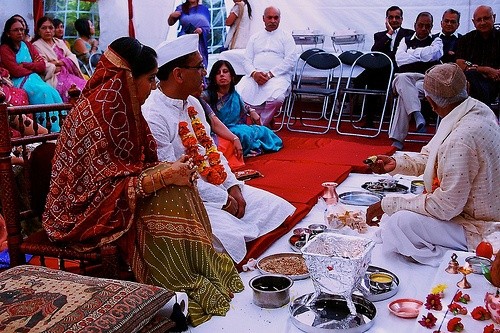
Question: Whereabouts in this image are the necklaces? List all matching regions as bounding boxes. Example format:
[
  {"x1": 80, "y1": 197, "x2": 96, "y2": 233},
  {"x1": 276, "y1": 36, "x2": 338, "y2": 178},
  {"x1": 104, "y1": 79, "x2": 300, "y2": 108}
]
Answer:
[{"x1": 179, "y1": 106, "x2": 228, "y2": 186}]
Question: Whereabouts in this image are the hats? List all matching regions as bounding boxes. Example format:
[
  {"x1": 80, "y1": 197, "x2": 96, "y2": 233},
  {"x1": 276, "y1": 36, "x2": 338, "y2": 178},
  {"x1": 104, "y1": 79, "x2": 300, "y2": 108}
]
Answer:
[
  {"x1": 423, "y1": 63, "x2": 466, "y2": 97},
  {"x1": 155, "y1": 33, "x2": 199, "y2": 67}
]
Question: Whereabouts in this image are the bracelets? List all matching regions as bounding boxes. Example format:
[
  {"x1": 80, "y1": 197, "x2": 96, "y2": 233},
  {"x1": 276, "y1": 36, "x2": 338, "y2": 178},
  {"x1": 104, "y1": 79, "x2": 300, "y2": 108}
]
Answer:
[
  {"x1": 158, "y1": 172, "x2": 166, "y2": 187},
  {"x1": 253, "y1": 72, "x2": 257, "y2": 78},
  {"x1": 223, "y1": 196, "x2": 231, "y2": 210},
  {"x1": 249, "y1": 109, "x2": 255, "y2": 112},
  {"x1": 231, "y1": 137, "x2": 239, "y2": 142},
  {"x1": 150, "y1": 174, "x2": 156, "y2": 196},
  {"x1": 139, "y1": 173, "x2": 151, "y2": 197},
  {"x1": 267, "y1": 73, "x2": 272, "y2": 79}
]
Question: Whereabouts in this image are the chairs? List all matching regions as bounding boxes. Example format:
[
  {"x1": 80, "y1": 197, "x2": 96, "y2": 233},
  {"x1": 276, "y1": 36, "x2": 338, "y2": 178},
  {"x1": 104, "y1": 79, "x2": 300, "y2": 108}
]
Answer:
[
  {"x1": 274, "y1": 49, "x2": 439, "y2": 144},
  {"x1": 0, "y1": 83, "x2": 102, "y2": 276}
]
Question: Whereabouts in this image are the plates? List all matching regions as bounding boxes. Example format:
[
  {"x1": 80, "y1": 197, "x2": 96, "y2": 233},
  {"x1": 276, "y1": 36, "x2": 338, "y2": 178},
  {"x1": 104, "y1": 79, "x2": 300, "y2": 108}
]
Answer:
[
  {"x1": 338, "y1": 193, "x2": 383, "y2": 206},
  {"x1": 256, "y1": 252, "x2": 310, "y2": 279}
]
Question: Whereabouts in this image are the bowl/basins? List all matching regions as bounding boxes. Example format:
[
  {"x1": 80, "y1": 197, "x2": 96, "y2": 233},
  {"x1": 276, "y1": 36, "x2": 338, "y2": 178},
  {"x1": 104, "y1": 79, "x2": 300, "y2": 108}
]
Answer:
[
  {"x1": 410, "y1": 179, "x2": 424, "y2": 195},
  {"x1": 378, "y1": 178, "x2": 399, "y2": 185},
  {"x1": 368, "y1": 271, "x2": 395, "y2": 291},
  {"x1": 249, "y1": 273, "x2": 295, "y2": 309},
  {"x1": 388, "y1": 298, "x2": 424, "y2": 318},
  {"x1": 368, "y1": 185, "x2": 384, "y2": 192},
  {"x1": 465, "y1": 256, "x2": 493, "y2": 274},
  {"x1": 289, "y1": 223, "x2": 328, "y2": 252}
]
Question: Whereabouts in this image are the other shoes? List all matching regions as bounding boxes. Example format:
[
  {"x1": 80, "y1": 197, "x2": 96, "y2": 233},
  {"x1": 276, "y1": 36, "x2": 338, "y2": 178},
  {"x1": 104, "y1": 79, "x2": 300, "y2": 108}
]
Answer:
[
  {"x1": 415, "y1": 120, "x2": 429, "y2": 134},
  {"x1": 341, "y1": 92, "x2": 350, "y2": 102},
  {"x1": 396, "y1": 146, "x2": 405, "y2": 151},
  {"x1": 363, "y1": 122, "x2": 375, "y2": 129}
]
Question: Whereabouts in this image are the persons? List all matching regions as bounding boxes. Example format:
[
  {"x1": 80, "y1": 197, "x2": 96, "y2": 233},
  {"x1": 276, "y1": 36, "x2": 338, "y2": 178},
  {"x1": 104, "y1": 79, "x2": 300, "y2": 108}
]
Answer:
[
  {"x1": 351, "y1": 5, "x2": 500, "y2": 150},
  {"x1": 201, "y1": 60, "x2": 283, "y2": 156},
  {"x1": 0, "y1": 14, "x2": 67, "y2": 133},
  {"x1": 53, "y1": 19, "x2": 104, "y2": 96},
  {"x1": 42, "y1": 36, "x2": 245, "y2": 328},
  {"x1": 365, "y1": 63, "x2": 500, "y2": 266},
  {"x1": 30, "y1": 16, "x2": 88, "y2": 103},
  {"x1": 234, "y1": 6, "x2": 297, "y2": 130},
  {"x1": 0, "y1": 68, "x2": 57, "y2": 235},
  {"x1": 190, "y1": 79, "x2": 264, "y2": 181},
  {"x1": 141, "y1": 33, "x2": 297, "y2": 264},
  {"x1": 168, "y1": 0, "x2": 253, "y2": 99}
]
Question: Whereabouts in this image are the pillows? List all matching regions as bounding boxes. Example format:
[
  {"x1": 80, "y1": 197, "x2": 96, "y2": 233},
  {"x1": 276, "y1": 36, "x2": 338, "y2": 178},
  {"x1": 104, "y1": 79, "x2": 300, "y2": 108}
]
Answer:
[{"x1": 0, "y1": 264, "x2": 189, "y2": 333}]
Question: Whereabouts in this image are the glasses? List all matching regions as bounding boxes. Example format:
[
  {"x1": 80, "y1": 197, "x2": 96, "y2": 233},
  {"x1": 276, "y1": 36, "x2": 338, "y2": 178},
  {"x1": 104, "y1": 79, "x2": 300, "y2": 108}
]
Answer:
[
  {"x1": 416, "y1": 22, "x2": 432, "y2": 29},
  {"x1": 387, "y1": 15, "x2": 402, "y2": 20},
  {"x1": 180, "y1": 59, "x2": 206, "y2": 71}
]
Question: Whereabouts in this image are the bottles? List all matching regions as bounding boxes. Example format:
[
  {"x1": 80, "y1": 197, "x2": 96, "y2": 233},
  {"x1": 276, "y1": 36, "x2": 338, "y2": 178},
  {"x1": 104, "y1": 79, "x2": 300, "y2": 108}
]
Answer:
[{"x1": 321, "y1": 182, "x2": 339, "y2": 206}]
